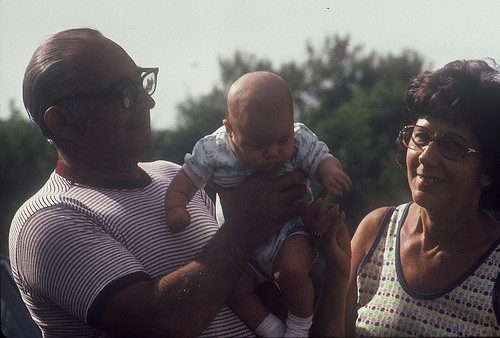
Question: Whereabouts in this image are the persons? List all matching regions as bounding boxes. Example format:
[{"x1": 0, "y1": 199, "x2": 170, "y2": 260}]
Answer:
[
  {"x1": 301, "y1": 60, "x2": 500, "y2": 338},
  {"x1": 8, "y1": 28, "x2": 308, "y2": 338},
  {"x1": 165, "y1": 71, "x2": 352, "y2": 338}
]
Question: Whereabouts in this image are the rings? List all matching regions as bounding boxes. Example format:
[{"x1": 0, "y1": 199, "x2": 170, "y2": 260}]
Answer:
[{"x1": 305, "y1": 228, "x2": 327, "y2": 240}]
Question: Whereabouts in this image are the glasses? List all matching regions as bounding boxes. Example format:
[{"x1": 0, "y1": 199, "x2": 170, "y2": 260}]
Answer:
[
  {"x1": 398, "y1": 124, "x2": 483, "y2": 161},
  {"x1": 54, "y1": 67, "x2": 158, "y2": 110}
]
